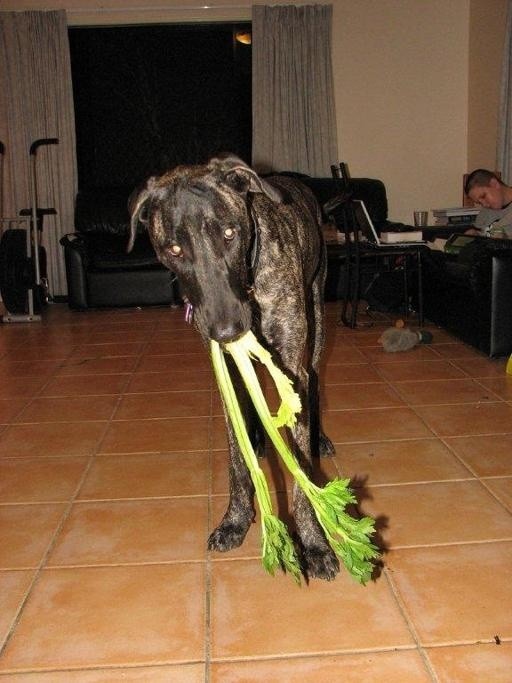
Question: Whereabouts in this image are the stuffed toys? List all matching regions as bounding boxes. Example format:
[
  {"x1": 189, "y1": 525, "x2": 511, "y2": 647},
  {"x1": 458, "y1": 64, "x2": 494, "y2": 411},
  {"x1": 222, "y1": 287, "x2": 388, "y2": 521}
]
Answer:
[{"x1": 377, "y1": 318, "x2": 432, "y2": 353}]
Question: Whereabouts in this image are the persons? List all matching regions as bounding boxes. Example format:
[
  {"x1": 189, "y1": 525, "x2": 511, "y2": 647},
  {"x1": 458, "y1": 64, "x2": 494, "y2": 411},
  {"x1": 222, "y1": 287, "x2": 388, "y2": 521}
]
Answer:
[{"x1": 464, "y1": 168, "x2": 511, "y2": 241}]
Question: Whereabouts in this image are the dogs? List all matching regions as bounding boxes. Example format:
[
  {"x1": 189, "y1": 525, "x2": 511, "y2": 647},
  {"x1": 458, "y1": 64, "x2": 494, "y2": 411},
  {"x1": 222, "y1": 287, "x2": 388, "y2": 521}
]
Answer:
[{"x1": 125, "y1": 151, "x2": 341, "y2": 582}]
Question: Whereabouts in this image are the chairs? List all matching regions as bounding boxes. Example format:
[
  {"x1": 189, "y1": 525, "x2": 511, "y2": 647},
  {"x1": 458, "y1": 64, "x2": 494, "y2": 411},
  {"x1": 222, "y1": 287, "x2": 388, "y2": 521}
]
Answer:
[
  {"x1": 406, "y1": 224, "x2": 512, "y2": 360},
  {"x1": 65, "y1": 189, "x2": 185, "y2": 312},
  {"x1": 301, "y1": 177, "x2": 415, "y2": 302},
  {"x1": 331, "y1": 162, "x2": 424, "y2": 329}
]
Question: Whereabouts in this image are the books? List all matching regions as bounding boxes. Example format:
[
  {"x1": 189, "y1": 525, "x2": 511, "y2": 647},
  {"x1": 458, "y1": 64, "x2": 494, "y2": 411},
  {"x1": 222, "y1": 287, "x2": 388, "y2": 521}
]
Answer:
[
  {"x1": 431, "y1": 206, "x2": 480, "y2": 225},
  {"x1": 433, "y1": 232, "x2": 491, "y2": 254},
  {"x1": 380, "y1": 229, "x2": 427, "y2": 243}
]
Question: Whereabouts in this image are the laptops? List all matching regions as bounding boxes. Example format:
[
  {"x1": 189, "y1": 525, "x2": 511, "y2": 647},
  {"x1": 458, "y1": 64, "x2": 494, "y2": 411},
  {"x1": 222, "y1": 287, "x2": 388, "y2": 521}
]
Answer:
[{"x1": 353, "y1": 200, "x2": 426, "y2": 247}]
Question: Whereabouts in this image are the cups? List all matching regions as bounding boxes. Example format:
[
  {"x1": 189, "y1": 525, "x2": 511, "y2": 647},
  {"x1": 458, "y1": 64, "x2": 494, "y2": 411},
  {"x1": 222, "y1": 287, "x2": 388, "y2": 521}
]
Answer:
[{"x1": 413, "y1": 211, "x2": 428, "y2": 227}]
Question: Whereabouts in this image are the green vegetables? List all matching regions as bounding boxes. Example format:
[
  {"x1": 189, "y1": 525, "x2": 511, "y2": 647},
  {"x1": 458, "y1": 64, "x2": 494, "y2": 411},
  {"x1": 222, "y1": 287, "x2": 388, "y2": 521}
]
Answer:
[{"x1": 210, "y1": 330, "x2": 378, "y2": 589}]
